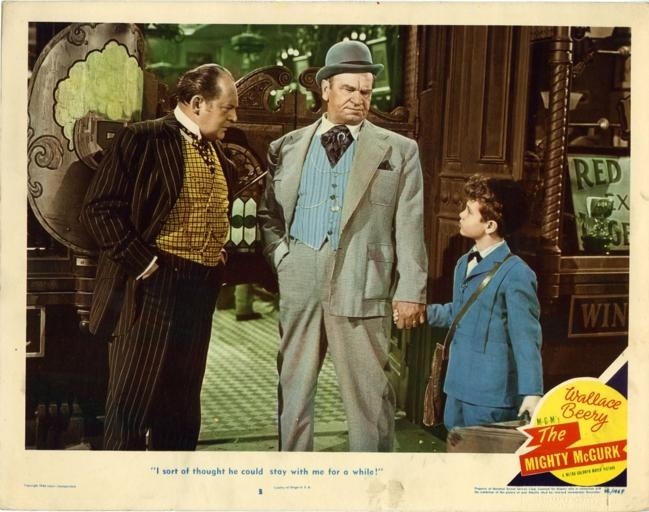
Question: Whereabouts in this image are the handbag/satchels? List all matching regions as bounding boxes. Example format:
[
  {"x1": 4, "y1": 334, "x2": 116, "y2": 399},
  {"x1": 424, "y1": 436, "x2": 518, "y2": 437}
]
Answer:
[{"x1": 422, "y1": 340, "x2": 449, "y2": 429}]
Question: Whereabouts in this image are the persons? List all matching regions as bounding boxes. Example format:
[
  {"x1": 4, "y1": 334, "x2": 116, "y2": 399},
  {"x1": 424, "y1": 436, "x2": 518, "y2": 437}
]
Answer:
[
  {"x1": 393, "y1": 170, "x2": 545, "y2": 432},
  {"x1": 76, "y1": 63, "x2": 240, "y2": 449},
  {"x1": 257, "y1": 40, "x2": 429, "y2": 450}
]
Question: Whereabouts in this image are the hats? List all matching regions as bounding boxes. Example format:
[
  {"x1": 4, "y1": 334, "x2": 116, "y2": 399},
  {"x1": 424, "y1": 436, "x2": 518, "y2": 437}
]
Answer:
[{"x1": 315, "y1": 40, "x2": 386, "y2": 88}]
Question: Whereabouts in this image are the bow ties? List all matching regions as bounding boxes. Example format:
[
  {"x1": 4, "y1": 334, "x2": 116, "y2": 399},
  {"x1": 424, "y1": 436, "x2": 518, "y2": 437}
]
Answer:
[
  {"x1": 192, "y1": 137, "x2": 215, "y2": 174},
  {"x1": 467, "y1": 251, "x2": 484, "y2": 265},
  {"x1": 320, "y1": 124, "x2": 355, "y2": 169}
]
